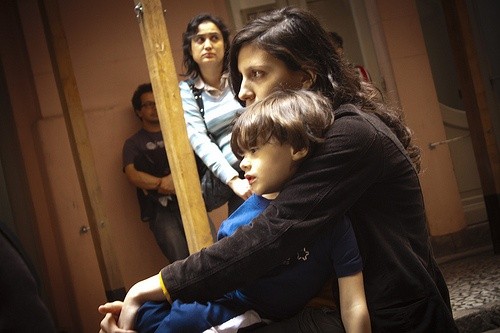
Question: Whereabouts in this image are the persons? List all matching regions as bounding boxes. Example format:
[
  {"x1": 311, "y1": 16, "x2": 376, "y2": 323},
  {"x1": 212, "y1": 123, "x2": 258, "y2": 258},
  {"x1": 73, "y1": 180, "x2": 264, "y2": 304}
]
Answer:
[
  {"x1": 122, "y1": 13, "x2": 373, "y2": 264},
  {"x1": 97, "y1": 7, "x2": 458, "y2": 333},
  {"x1": 131, "y1": 89, "x2": 372, "y2": 333}
]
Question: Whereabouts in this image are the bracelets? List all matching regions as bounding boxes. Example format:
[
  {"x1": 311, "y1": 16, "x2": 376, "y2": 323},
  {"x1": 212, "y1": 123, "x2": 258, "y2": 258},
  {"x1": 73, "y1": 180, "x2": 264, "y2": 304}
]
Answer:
[{"x1": 155, "y1": 177, "x2": 163, "y2": 192}]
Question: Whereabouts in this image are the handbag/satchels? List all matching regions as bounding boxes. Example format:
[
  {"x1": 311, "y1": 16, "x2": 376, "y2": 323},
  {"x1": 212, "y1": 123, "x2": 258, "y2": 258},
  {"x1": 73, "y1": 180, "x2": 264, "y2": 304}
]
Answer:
[{"x1": 192, "y1": 131, "x2": 237, "y2": 212}]
